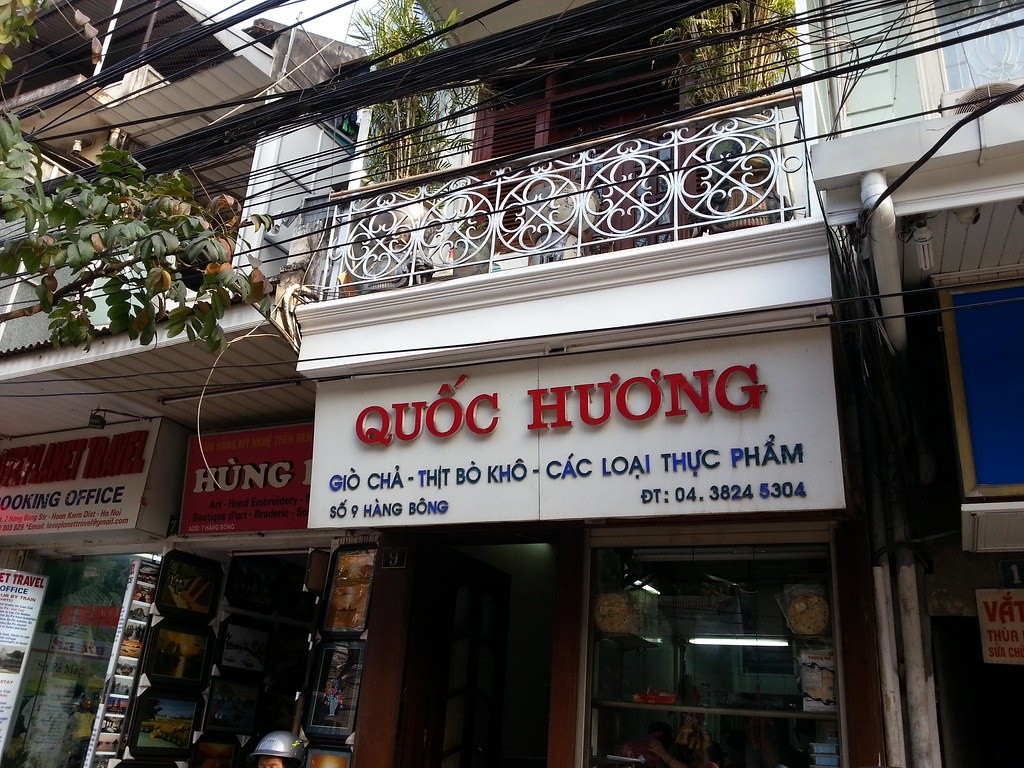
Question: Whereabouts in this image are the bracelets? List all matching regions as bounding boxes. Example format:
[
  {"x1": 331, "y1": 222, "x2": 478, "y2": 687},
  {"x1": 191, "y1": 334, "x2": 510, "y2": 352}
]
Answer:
[{"x1": 666, "y1": 757, "x2": 674, "y2": 764}]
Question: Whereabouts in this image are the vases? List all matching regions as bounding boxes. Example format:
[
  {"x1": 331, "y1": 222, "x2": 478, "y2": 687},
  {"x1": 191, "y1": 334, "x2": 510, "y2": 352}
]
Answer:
[
  {"x1": 528, "y1": 179, "x2": 602, "y2": 241},
  {"x1": 446, "y1": 227, "x2": 492, "y2": 275}
]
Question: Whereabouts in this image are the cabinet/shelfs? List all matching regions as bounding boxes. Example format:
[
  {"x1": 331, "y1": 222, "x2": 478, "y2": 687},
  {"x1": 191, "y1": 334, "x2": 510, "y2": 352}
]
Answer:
[{"x1": 573, "y1": 520, "x2": 854, "y2": 768}]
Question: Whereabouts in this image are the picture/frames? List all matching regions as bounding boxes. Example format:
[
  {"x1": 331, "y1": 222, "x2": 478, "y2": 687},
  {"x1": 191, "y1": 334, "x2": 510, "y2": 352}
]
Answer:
[
  {"x1": 211, "y1": 610, "x2": 276, "y2": 686},
  {"x1": 122, "y1": 761, "x2": 192, "y2": 768},
  {"x1": 314, "y1": 542, "x2": 382, "y2": 643},
  {"x1": 140, "y1": 617, "x2": 220, "y2": 691},
  {"x1": 199, "y1": 678, "x2": 262, "y2": 735},
  {"x1": 147, "y1": 544, "x2": 228, "y2": 625},
  {"x1": 300, "y1": 739, "x2": 354, "y2": 768},
  {"x1": 121, "y1": 691, "x2": 209, "y2": 764},
  {"x1": 299, "y1": 642, "x2": 368, "y2": 746},
  {"x1": 190, "y1": 730, "x2": 252, "y2": 768}
]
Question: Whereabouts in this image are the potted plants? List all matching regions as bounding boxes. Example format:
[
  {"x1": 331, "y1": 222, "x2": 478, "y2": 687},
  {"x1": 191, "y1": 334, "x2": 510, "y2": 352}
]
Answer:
[
  {"x1": 648, "y1": 0, "x2": 797, "y2": 177},
  {"x1": 337, "y1": 0, "x2": 513, "y2": 253}
]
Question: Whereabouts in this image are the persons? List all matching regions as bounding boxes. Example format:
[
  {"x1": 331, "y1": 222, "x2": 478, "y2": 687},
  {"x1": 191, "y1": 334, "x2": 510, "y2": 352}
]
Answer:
[
  {"x1": 69, "y1": 699, "x2": 95, "y2": 746},
  {"x1": 250, "y1": 730, "x2": 305, "y2": 768},
  {"x1": 616, "y1": 720, "x2": 718, "y2": 768}
]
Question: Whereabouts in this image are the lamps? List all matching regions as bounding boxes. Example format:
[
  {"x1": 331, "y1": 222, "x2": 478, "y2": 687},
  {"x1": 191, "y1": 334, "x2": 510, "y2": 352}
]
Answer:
[
  {"x1": 913, "y1": 216, "x2": 935, "y2": 270},
  {"x1": 88, "y1": 407, "x2": 143, "y2": 430},
  {"x1": 72, "y1": 140, "x2": 82, "y2": 152}
]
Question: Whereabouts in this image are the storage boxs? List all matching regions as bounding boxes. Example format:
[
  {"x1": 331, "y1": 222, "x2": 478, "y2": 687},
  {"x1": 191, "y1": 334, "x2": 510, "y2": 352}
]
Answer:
[
  {"x1": 809, "y1": 743, "x2": 839, "y2": 755},
  {"x1": 810, "y1": 754, "x2": 840, "y2": 766}
]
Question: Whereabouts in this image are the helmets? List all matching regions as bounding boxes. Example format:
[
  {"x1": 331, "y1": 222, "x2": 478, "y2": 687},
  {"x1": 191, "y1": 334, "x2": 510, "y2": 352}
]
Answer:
[{"x1": 250, "y1": 730, "x2": 304, "y2": 767}]
